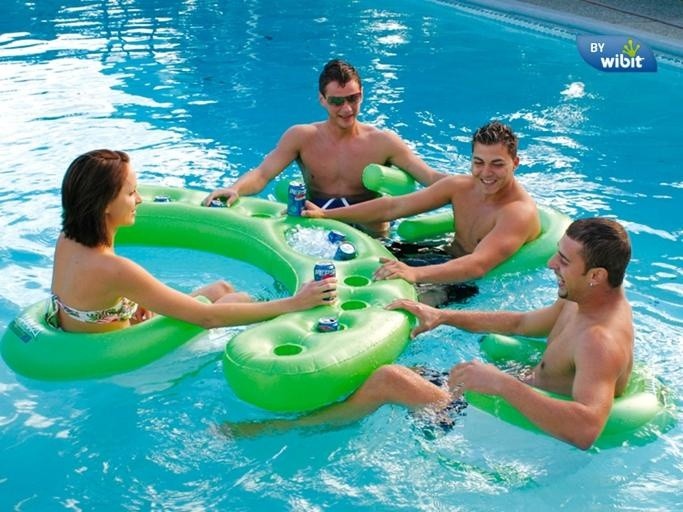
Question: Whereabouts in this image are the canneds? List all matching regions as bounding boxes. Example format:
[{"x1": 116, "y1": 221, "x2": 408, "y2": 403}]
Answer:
[
  {"x1": 210, "y1": 199, "x2": 226, "y2": 207},
  {"x1": 155, "y1": 194, "x2": 172, "y2": 203},
  {"x1": 317, "y1": 317, "x2": 339, "y2": 333},
  {"x1": 288, "y1": 179, "x2": 308, "y2": 216},
  {"x1": 312, "y1": 262, "x2": 336, "y2": 302},
  {"x1": 334, "y1": 242, "x2": 355, "y2": 262},
  {"x1": 328, "y1": 230, "x2": 346, "y2": 241}
]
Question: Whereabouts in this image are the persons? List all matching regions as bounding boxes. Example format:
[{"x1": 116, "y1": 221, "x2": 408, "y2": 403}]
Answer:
[
  {"x1": 202, "y1": 61, "x2": 451, "y2": 240},
  {"x1": 281, "y1": 122, "x2": 541, "y2": 281},
  {"x1": 219, "y1": 217, "x2": 635, "y2": 451},
  {"x1": 51, "y1": 149, "x2": 337, "y2": 336}
]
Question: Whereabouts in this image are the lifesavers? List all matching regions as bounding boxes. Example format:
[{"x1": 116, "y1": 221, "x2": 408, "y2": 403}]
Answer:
[
  {"x1": 112, "y1": 184, "x2": 417, "y2": 413},
  {"x1": 461, "y1": 332, "x2": 677, "y2": 453},
  {"x1": 277, "y1": 162, "x2": 419, "y2": 204},
  {"x1": 1, "y1": 296, "x2": 212, "y2": 380},
  {"x1": 397, "y1": 203, "x2": 573, "y2": 278}
]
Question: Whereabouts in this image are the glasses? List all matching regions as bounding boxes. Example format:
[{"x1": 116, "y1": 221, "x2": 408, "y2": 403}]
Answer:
[{"x1": 326, "y1": 92, "x2": 362, "y2": 106}]
